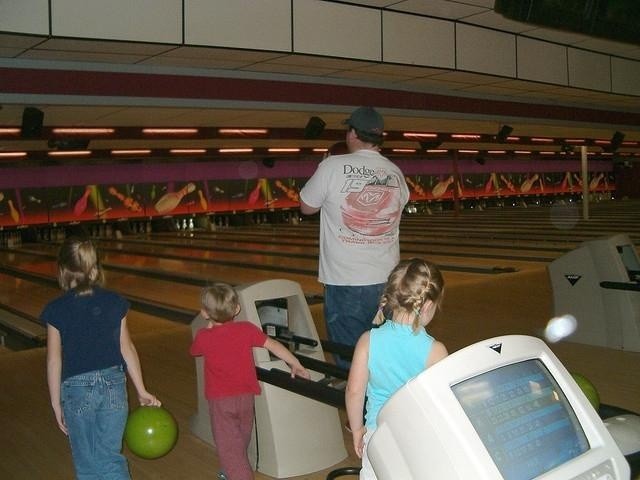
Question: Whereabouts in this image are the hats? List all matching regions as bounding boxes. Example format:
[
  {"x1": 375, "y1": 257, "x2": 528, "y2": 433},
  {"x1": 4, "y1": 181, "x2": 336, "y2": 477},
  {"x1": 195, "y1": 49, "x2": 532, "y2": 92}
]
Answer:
[{"x1": 341, "y1": 106, "x2": 385, "y2": 137}]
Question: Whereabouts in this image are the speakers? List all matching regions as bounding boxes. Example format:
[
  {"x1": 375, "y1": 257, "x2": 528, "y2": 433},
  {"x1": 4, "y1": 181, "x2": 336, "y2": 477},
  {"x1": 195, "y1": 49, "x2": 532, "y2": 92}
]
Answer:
[
  {"x1": 611, "y1": 131, "x2": 626, "y2": 145},
  {"x1": 21, "y1": 107, "x2": 44, "y2": 138},
  {"x1": 498, "y1": 125, "x2": 513, "y2": 137},
  {"x1": 304, "y1": 116, "x2": 326, "y2": 137}
]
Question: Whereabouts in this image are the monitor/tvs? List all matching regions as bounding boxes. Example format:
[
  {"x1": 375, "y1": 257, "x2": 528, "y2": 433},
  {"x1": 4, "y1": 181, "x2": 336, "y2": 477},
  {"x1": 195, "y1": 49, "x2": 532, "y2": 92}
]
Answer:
[{"x1": 450, "y1": 358, "x2": 590, "y2": 480}]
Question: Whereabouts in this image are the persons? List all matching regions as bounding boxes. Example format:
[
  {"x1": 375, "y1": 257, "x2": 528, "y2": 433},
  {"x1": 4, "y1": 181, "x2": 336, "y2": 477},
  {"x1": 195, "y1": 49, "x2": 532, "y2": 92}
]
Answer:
[
  {"x1": 188, "y1": 283, "x2": 311, "y2": 479},
  {"x1": 40, "y1": 237, "x2": 162, "y2": 480},
  {"x1": 298, "y1": 107, "x2": 411, "y2": 432},
  {"x1": 344, "y1": 256, "x2": 449, "y2": 480}
]
